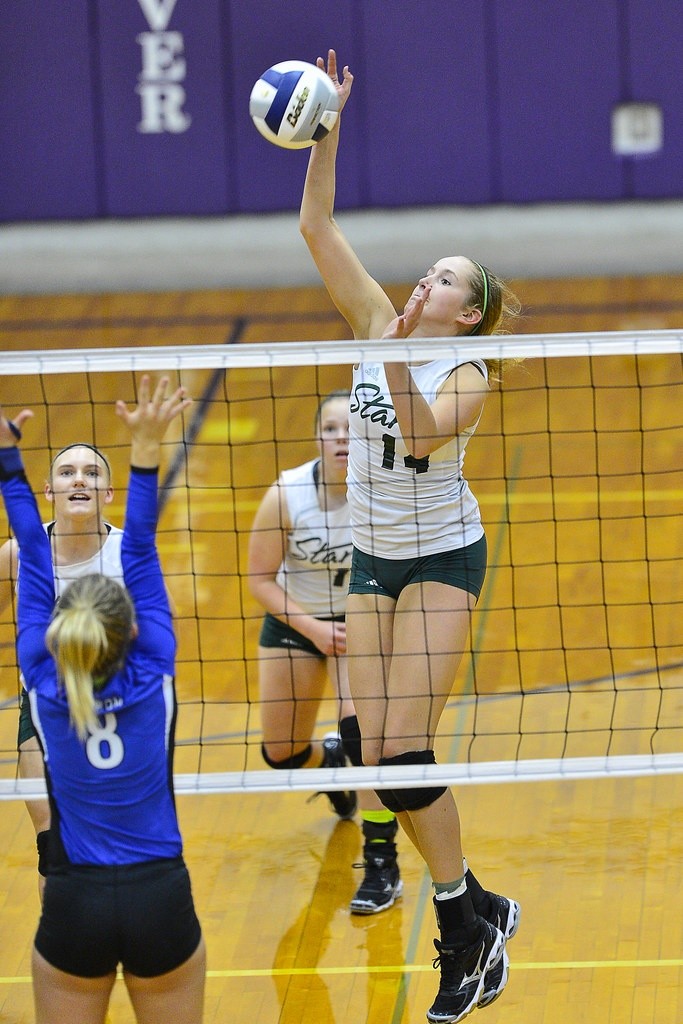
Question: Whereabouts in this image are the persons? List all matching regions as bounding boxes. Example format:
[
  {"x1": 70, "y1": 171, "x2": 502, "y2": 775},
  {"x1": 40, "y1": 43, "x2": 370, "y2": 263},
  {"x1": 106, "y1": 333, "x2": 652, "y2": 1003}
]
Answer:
[
  {"x1": 0, "y1": 371, "x2": 206, "y2": 1023},
  {"x1": 2, "y1": 442, "x2": 127, "y2": 913},
  {"x1": 297, "y1": 46, "x2": 524, "y2": 1024},
  {"x1": 243, "y1": 388, "x2": 407, "y2": 917}
]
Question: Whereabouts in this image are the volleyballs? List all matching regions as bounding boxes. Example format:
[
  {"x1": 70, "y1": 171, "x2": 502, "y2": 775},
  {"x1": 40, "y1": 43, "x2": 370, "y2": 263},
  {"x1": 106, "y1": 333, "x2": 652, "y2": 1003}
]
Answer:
[{"x1": 247, "y1": 59, "x2": 342, "y2": 152}]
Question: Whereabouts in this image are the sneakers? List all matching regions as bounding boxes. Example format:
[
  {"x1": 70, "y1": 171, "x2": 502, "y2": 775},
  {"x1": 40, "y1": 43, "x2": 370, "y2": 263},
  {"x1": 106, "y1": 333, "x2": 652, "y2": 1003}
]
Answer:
[
  {"x1": 475, "y1": 890, "x2": 520, "y2": 1009},
  {"x1": 349, "y1": 846, "x2": 402, "y2": 914},
  {"x1": 426, "y1": 913, "x2": 506, "y2": 1024},
  {"x1": 306, "y1": 732, "x2": 357, "y2": 820}
]
